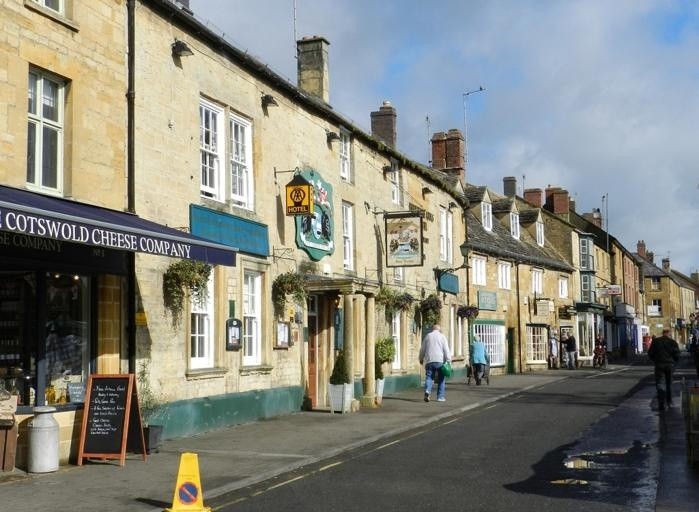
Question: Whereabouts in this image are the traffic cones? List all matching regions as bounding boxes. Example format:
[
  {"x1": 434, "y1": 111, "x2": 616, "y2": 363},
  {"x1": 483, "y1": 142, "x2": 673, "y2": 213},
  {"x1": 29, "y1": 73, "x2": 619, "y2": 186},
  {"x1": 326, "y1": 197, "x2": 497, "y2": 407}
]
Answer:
[{"x1": 164, "y1": 452, "x2": 212, "y2": 512}]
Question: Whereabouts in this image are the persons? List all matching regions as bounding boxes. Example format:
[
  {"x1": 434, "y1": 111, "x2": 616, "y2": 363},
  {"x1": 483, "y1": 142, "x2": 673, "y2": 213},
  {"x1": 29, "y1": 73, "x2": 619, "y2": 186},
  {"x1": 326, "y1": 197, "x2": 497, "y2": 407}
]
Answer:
[
  {"x1": 469, "y1": 335, "x2": 489, "y2": 385},
  {"x1": 416, "y1": 324, "x2": 453, "y2": 403},
  {"x1": 648, "y1": 327, "x2": 680, "y2": 410},
  {"x1": 593, "y1": 345, "x2": 605, "y2": 365},
  {"x1": 594, "y1": 332, "x2": 609, "y2": 347},
  {"x1": 548, "y1": 330, "x2": 581, "y2": 370}
]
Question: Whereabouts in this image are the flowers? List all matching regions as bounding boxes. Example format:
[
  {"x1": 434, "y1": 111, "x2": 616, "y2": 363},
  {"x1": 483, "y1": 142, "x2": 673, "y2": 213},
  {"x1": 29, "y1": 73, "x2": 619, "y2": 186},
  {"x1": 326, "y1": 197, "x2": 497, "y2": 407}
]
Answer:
[{"x1": 376, "y1": 287, "x2": 479, "y2": 326}]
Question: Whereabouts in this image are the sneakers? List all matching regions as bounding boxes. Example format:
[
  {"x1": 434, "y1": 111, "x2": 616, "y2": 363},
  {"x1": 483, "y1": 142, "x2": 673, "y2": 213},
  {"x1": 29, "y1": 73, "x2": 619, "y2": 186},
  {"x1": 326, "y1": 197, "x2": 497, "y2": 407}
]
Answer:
[
  {"x1": 424, "y1": 390, "x2": 430, "y2": 402},
  {"x1": 437, "y1": 398, "x2": 446, "y2": 402}
]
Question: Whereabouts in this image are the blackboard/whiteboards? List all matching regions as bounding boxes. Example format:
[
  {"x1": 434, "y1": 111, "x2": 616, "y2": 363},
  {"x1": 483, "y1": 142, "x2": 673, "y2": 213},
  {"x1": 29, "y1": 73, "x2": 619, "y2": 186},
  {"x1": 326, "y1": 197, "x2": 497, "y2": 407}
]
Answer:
[
  {"x1": 68, "y1": 383, "x2": 85, "y2": 403},
  {"x1": 79, "y1": 374, "x2": 134, "y2": 460}
]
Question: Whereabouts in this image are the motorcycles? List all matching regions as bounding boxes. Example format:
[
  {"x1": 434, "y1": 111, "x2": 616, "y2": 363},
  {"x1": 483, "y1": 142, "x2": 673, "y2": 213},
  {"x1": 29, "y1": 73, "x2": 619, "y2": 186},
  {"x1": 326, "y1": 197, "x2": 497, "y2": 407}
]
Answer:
[{"x1": 593, "y1": 345, "x2": 607, "y2": 370}]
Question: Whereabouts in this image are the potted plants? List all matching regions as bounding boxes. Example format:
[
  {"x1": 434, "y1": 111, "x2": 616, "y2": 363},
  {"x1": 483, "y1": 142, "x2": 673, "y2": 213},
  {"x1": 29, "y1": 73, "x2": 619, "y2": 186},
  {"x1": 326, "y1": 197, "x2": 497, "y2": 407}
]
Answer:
[
  {"x1": 164, "y1": 260, "x2": 211, "y2": 330},
  {"x1": 374, "y1": 348, "x2": 385, "y2": 406},
  {"x1": 330, "y1": 348, "x2": 351, "y2": 413},
  {"x1": 273, "y1": 271, "x2": 306, "y2": 317}
]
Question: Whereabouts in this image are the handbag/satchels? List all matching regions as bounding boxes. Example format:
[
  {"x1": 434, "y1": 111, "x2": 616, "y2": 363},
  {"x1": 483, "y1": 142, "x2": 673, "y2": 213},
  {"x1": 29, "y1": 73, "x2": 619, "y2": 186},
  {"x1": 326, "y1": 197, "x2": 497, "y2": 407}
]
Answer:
[{"x1": 441, "y1": 363, "x2": 452, "y2": 378}]
